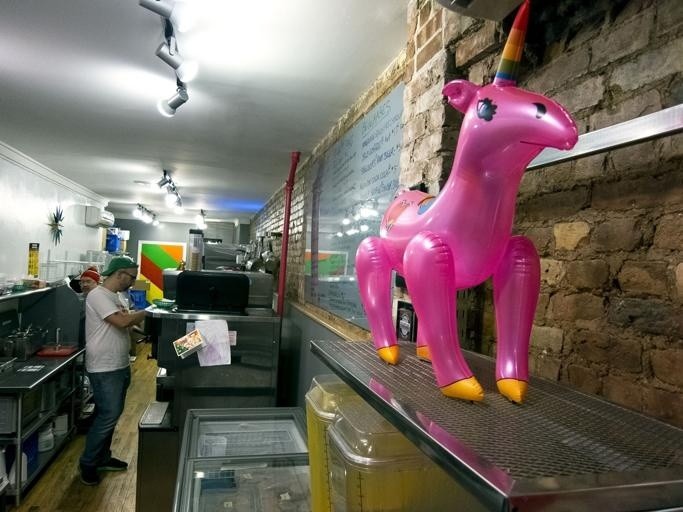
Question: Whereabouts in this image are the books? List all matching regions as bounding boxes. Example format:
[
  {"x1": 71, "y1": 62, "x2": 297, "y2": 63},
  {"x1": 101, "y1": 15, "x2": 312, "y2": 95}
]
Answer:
[{"x1": 170, "y1": 329, "x2": 207, "y2": 358}]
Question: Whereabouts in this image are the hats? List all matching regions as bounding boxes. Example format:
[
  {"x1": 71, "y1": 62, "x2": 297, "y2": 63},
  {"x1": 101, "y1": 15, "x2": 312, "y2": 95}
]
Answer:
[
  {"x1": 101, "y1": 256, "x2": 138, "y2": 277},
  {"x1": 81, "y1": 266, "x2": 100, "y2": 283}
]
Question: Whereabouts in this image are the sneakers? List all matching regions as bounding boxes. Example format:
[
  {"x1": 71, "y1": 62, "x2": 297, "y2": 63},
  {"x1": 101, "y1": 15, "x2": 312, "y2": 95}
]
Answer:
[
  {"x1": 77, "y1": 461, "x2": 99, "y2": 486},
  {"x1": 97, "y1": 457, "x2": 129, "y2": 472}
]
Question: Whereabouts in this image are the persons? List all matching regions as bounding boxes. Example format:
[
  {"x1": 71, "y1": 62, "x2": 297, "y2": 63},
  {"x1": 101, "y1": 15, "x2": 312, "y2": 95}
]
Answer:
[
  {"x1": 78, "y1": 251, "x2": 156, "y2": 485},
  {"x1": 71, "y1": 266, "x2": 99, "y2": 299}
]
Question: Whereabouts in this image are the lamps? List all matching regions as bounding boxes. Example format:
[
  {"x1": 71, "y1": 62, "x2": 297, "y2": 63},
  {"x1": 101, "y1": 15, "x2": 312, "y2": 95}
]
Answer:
[
  {"x1": 133, "y1": 205, "x2": 157, "y2": 227},
  {"x1": 138, "y1": 1, "x2": 189, "y2": 119},
  {"x1": 157, "y1": 171, "x2": 182, "y2": 208}
]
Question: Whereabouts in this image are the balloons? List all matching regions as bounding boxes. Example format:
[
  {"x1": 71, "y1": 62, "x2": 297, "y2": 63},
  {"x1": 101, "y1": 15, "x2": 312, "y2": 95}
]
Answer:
[{"x1": 354, "y1": 1, "x2": 582, "y2": 407}]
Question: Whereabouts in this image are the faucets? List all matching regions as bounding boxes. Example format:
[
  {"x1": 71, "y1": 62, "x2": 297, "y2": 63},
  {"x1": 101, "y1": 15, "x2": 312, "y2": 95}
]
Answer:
[{"x1": 54, "y1": 328, "x2": 64, "y2": 351}]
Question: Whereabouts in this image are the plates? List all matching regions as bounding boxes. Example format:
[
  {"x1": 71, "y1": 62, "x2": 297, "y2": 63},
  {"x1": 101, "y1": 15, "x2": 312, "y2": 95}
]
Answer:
[{"x1": 151, "y1": 298, "x2": 176, "y2": 308}]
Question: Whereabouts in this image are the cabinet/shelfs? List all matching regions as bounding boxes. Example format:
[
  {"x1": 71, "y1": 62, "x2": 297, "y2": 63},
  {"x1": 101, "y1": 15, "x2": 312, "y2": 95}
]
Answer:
[
  {"x1": 45, "y1": 250, "x2": 105, "y2": 297},
  {"x1": 0, "y1": 284, "x2": 88, "y2": 512}
]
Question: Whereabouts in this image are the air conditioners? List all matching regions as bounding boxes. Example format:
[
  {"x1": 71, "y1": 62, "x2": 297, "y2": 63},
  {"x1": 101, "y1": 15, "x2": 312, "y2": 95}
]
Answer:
[{"x1": 85, "y1": 206, "x2": 115, "y2": 228}]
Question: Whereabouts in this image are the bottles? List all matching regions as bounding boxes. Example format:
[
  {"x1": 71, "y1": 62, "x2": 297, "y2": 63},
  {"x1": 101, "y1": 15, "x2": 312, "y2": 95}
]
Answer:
[
  {"x1": 27, "y1": 242, "x2": 39, "y2": 277},
  {"x1": 188, "y1": 228, "x2": 203, "y2": 270}
]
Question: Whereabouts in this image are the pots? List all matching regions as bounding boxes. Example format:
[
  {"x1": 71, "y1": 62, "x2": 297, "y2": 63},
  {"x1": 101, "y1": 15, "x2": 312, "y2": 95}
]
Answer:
[{"x1": 2, "y1": 333, "x2": 32, "y2": 362}]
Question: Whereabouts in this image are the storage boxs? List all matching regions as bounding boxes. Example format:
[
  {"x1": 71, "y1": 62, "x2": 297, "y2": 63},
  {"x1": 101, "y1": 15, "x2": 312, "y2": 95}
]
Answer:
[
  {"x1": 330, "y1": 404, "x2": 484, "y2": 512},
  {"x1": 119, "y1": 241, "x2": 126, "y2": 251},
  {"x1": 106, "y1": 234, "x2": 120, "y2": 252},
  {"x1": 305, "y1": 373, "x2": 364, "y2": 512},
  {"x1": 130, "y1": 289, "x2": 146, "y2": 309}
]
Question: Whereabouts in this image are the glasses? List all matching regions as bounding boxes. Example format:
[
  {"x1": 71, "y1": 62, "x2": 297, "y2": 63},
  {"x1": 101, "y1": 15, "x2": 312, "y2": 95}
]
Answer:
[{"x1": 121, "y1": 270, "x2": 137, "y2": 280}]
